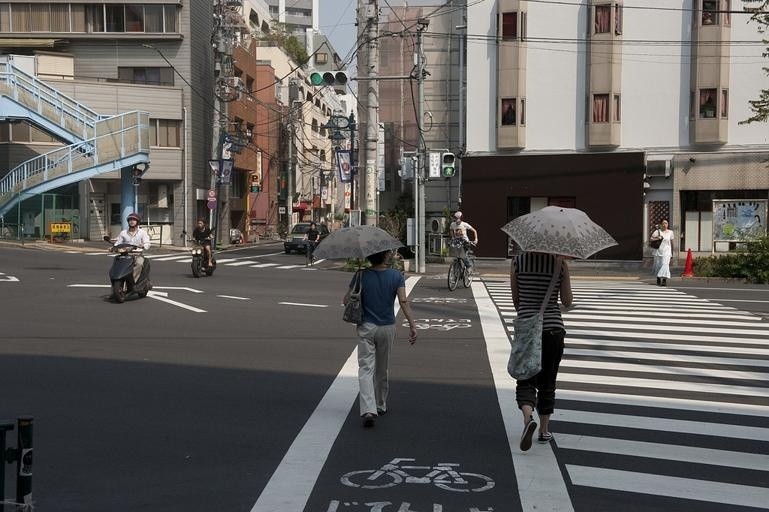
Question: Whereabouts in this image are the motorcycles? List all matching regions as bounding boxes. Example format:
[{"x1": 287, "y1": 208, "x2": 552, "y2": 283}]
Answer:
[
  {"x1": 106, "y1": 243, "x2": 162, "y2": 307},
  {"x1": 182, "y1": 236, "x2": 219, "y2": 280}
]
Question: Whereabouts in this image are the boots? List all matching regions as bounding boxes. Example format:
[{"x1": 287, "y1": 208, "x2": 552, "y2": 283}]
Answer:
[
  {"x1": 662, "y1": 279, "x2": 666, "y2": 286},
  {"x1": 657, "y1": 278, "x2": 661, "y2": 285}
]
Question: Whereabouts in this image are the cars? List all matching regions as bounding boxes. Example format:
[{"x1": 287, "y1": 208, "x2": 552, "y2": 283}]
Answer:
[{"x1": 285, "y1": 219, "x2": 334, "y2": 257}]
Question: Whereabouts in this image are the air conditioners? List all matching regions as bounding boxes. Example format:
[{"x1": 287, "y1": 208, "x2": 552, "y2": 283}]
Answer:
[{"x1": 428, "y1": 216, "x2": 447, "y2": 233}]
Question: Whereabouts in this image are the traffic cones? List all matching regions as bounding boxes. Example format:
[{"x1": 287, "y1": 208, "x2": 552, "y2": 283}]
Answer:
[{"x1": 678, "y1": 248, "x2": 696, "y2": 281}]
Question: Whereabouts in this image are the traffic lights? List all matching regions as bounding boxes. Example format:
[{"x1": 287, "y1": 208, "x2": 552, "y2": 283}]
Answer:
[
  {"x1": 308, "y1": 66, "x2": 350, "y2": 89},
  {"x1": 441, "y1": 150, "x2": 462, "y2": 184}
]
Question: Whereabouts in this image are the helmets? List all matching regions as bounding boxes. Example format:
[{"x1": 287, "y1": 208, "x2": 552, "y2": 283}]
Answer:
[{"x1": 127, "y1": 214, "x2": 140, "y2": 221}]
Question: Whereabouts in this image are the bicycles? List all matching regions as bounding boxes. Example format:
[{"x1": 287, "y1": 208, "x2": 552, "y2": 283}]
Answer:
[
  {"x1": 303, "y1": 236, "x2": 323, "y2": 262},
  {"x1": 445, "y1": 253, "x2": 483, "y2": 296}
]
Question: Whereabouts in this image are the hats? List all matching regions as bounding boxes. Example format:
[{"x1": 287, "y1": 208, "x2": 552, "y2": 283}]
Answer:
[{"x1": 453, "y1": 212, "x2": 463, "y2": 219}]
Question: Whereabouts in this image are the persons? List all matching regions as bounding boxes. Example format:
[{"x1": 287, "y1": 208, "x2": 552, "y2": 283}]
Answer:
[
  {"x1": 648, "y1": 216, "x2": 677, "y2": 286},
  {"x1": 107, "y1": 213, "x2": 150, "y2": 281},
  {"x1": 302, "y1": 220, "x2": 321, "y2": 258},
  {"x1": 507, "y1": 246, "x2": 574, "y2": 452},
  {"x1": 188, "y1": 215, "x2": 216, "y2": 268},
  {"x1": 447, "y1": 210, "x2": 479, "y2": 273},
  {"x1": 341, "y1": 247, "x2": 420, "y2": 426}
]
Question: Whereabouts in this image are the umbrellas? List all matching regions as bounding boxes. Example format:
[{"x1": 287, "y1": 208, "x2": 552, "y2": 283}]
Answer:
[
  {"x1": 312, "y1": 223, "x2": 407, "y2": 263},
  {"x1": 501, "y1": 199, "x2": 620, "y2": 261}
]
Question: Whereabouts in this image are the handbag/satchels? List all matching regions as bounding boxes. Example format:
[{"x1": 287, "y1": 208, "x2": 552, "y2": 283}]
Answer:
[
  {"x1": 651, "y1": 229, "x2": 663, "y2": 249},
  {"x1": 508, "y1": 313, "x2": 543, "y2": 380},
  {"x1": 343, "y1": 269, "x2": 364, "y2": 324}
]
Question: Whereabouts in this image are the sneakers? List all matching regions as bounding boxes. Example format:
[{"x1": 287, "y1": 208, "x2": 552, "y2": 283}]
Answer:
[
  {"x1": 377, "y1": 409, "x2": 384, "y2": 415},
  {"x1": 520, "y1": 419, "x2": 537, "y2": 451},
  {"x1": 363, "y1": 413, "x2": 376, "y2": 428},
  {"x1": 538, "y1": 432, "x2": 553, "y2": 443}
]
